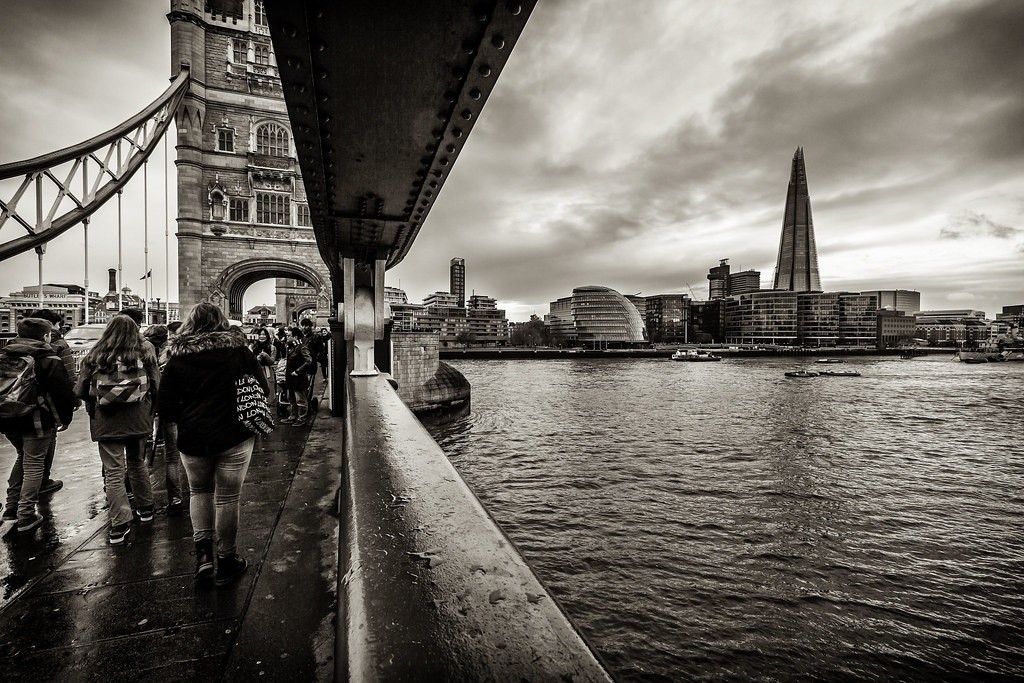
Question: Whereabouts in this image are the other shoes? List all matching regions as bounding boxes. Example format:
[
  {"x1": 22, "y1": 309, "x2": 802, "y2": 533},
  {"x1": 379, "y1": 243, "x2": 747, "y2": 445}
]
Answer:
[
  {"x1": 151, "y1": 478, "x2": 168, "y2": 493},
  {"x1": 40, "y1": 480, "x2": 63, "y2": 493}
]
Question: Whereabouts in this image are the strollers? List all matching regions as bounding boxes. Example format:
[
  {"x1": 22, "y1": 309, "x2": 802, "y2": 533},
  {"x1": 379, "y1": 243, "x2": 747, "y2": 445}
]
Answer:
[{"x1": 275, "y1": 356, "x2": 318, "y2": 416}]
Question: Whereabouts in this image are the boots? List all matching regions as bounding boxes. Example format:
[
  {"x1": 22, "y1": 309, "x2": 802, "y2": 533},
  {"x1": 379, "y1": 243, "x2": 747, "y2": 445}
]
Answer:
[
  {"x1": 193, "y1": 546, "x2": 215, "y2": 579},
  {"x1": 215, "y1": 555, "x2": 247, "y2": 585}
]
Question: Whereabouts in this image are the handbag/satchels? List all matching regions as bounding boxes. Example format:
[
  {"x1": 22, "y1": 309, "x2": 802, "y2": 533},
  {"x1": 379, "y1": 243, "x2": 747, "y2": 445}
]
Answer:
[{"x1": 235, "y1": 375, "x2": 275, "y2": 439}]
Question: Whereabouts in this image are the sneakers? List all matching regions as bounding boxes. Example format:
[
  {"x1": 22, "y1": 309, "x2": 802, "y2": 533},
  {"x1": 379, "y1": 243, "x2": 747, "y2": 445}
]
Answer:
[
  {"x1": 281, "y1": 415, "x2": 296, "y2": 424},
  {"x1": 292, "y1": 419, "x2": 306, "y2": 426},
  {"x1": 109, "y1": 527, "x2": 130, "y2": 543},
  {"x1": 137, "y1": 507, "x2": 154, "y2": 521},
  {"x1": 2, "y1": 508, "x2": 18, "y2": 519},
  {"x1": 17, "y1": 514, "x2": 43, "y2": 531}
]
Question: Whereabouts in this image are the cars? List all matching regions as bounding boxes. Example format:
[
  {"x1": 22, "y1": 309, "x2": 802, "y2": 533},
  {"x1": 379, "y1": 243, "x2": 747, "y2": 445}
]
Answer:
[{"x1": 63, "y1": 324, "x2": 108, "y2": 365}]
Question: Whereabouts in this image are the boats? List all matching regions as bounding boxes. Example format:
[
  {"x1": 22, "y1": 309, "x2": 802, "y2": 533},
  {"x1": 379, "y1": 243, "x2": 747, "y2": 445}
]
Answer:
[
  {"x1": 815, "y1": 359, "x2": 846, "y2": 363},
  {"x1": 958, "y1": 327, "x2": 1024, "y2": 363},
  {"x1": 671, "y1": 347, "x2": 722, "y2": 361},
  {"x1": 784, "y1": 367, "x2": 860, "y2": 377}
]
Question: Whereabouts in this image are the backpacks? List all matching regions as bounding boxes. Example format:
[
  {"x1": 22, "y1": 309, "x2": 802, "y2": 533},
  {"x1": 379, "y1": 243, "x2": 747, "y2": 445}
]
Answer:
[
  {"x1": 96, "y1": 358, "x2": 150, "y2": 404},
  {"x1": 0, "y1": 350, "x2": 58, "y2": 418}
]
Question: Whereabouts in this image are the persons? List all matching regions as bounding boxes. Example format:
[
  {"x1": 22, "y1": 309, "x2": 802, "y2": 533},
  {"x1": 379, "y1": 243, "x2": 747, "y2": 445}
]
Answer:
[
  {"x1": 0, "y1": 301, "x2": 334, "y2": 581},
  {"x1": 0, "y1": 317, "x2": 73, "y2": 532},
  {"x1": 158, "y1": 301, "x2": 271, "y2": 581},
  {"x1": 74, "y1": 314, "x2": 162, "y2": 545}
]
